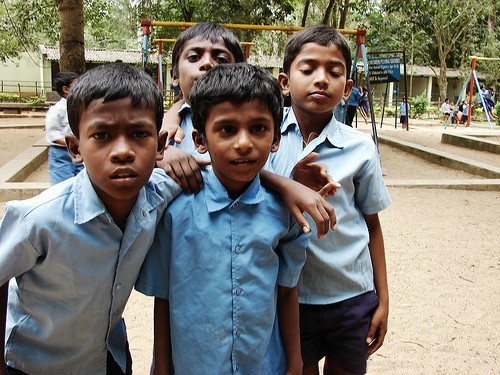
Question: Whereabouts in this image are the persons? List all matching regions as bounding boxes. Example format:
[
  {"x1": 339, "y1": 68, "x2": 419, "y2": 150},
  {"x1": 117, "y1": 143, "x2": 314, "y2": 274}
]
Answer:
[
  {"x1": 43, "y1": 72, "x2": 84, "y2": 186},
  {"x1": 158, "y1": 25, "x2": 390, "y2": 375},
  {"x1": 156, "y1": 21, "x2": 342, "y2": 197},
  {"x1": 334, "y1": 78, "x2": 370, "y2": 127},
  {"x1": 133, "y1": 64, "x2": 311, "y2": 375},
  {"x1": 441, "y1": 84, "x2": 497, "y2": 124},
  {"x1": 170, "y1": 65, "x2": 183, "y2": 104},
  {"x1": 0, "y1": 62, "x2": 337, "y2": 375},
  {"x1": 400, "y1": 96, "x2": 410, "y2": 129}
]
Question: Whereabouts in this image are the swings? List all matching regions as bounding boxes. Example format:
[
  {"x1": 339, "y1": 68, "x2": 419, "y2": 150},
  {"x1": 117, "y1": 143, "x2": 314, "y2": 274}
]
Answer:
[{"x1": 479, "y1": 61, "x2": 500, "y2": 107}]
